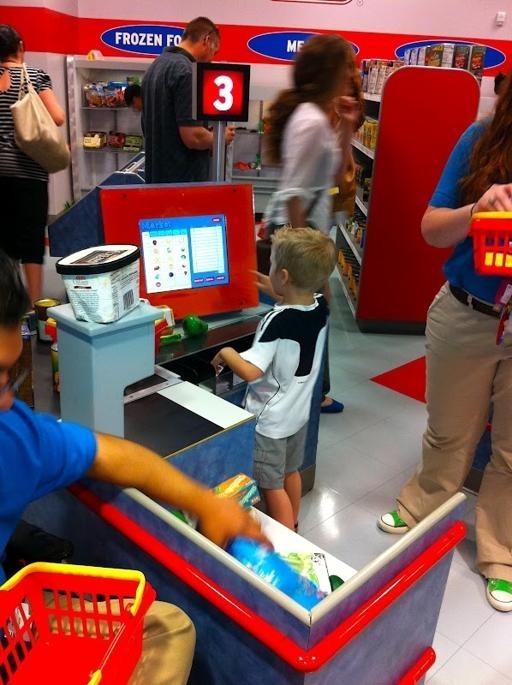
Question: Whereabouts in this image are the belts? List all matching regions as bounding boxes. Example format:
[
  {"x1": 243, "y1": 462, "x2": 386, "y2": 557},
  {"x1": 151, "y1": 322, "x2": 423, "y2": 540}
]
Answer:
[{"x1": 447, "y1": 282, "x2": 511, "y2": 320}]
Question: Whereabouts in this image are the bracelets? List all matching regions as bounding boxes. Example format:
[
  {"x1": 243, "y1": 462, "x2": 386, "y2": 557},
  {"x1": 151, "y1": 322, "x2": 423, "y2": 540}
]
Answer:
[{"x1": 471, "y1": 203, "x2": 476, "y2": 217}]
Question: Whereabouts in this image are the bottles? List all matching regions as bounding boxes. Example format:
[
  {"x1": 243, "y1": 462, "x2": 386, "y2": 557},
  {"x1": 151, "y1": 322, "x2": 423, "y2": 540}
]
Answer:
[
  {"x1": 35, "y1": 298, "x2": 62, "y2": 343},
  {"x1": 50, "y1": 343, "x2": 60, "y2": 393}
]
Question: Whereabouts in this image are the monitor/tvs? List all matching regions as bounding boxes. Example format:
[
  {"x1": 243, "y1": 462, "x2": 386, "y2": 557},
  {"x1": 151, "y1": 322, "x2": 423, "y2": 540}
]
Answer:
[{"x1": 97, "y1": 183, "x2": 259, "y2": 319}]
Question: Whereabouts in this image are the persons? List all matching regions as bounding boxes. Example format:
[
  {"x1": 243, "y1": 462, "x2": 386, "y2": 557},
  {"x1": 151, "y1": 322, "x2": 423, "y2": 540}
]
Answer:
[
  {"x1": 213, "y1": 228, "x2": 336, "y2": 533},
  {"x1": 0, "y1": 21, "x2": 66, "y2": 311},
  {"x1": 379, "y1": 70, "x2": 512, "y2": 611},
  {"x1": 140, "y1": 17, "x2": 235, "y2": 184},
  {"x1": 0, "y1": 251, "x2": 274, "y2": 685},
  {"x1": 252, "y1": 35, "x2": 359, "y2": 414}
]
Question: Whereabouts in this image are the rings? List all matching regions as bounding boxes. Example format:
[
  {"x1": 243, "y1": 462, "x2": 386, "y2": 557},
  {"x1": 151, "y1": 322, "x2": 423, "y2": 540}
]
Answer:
[{"x1": 488, "y1": 194, "x2": 498, "y2": 205}]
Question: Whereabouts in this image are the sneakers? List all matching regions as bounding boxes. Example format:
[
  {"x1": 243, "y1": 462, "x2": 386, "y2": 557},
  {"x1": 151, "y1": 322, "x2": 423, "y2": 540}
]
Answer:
[
  {"x1": 485, "y1": 576, "x2": 512, "y2": 610},
  {"x1": 378, "y1": 508, "x2": 409, "y2": 533}
]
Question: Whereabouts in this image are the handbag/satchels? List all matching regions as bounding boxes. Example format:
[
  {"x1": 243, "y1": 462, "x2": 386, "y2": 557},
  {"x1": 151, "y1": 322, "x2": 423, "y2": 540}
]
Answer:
[
  {"x1": 10, "y1": 64, "x2": 71, "y2": 174},
  {"x1": 257, "y1": 148, "x2": 332, "y2": 274}
]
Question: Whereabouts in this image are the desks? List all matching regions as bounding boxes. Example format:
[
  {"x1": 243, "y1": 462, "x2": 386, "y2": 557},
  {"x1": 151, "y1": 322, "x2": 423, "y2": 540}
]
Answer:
[
  {"x1": 51, "y1": 364, "x2": 471, "y2": 685},
  {"x1": 154, "y1": 290, "x2": 326, "y2": 499}
]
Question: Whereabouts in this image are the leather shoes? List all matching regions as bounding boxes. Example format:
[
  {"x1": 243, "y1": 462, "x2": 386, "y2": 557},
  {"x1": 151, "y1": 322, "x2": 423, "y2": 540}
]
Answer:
[{"x1": 320, "y1": 397, "x2": 344, "y2": 413}]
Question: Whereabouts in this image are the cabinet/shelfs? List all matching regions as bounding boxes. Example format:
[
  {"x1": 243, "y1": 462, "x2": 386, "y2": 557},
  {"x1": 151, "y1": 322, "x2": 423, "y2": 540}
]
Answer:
[
  {"x1": 335, "y1": 65, "x2": 481, "y2": 335},
  {"x1": 65, "y1": 51, "x2": 156, "y2": 204},
  {"x1": 23, "y1": 50, "x2": 70, "y2": 218},
  {"x1": 220, "y1": 98, "x2": 283, "y2": 217}
]
